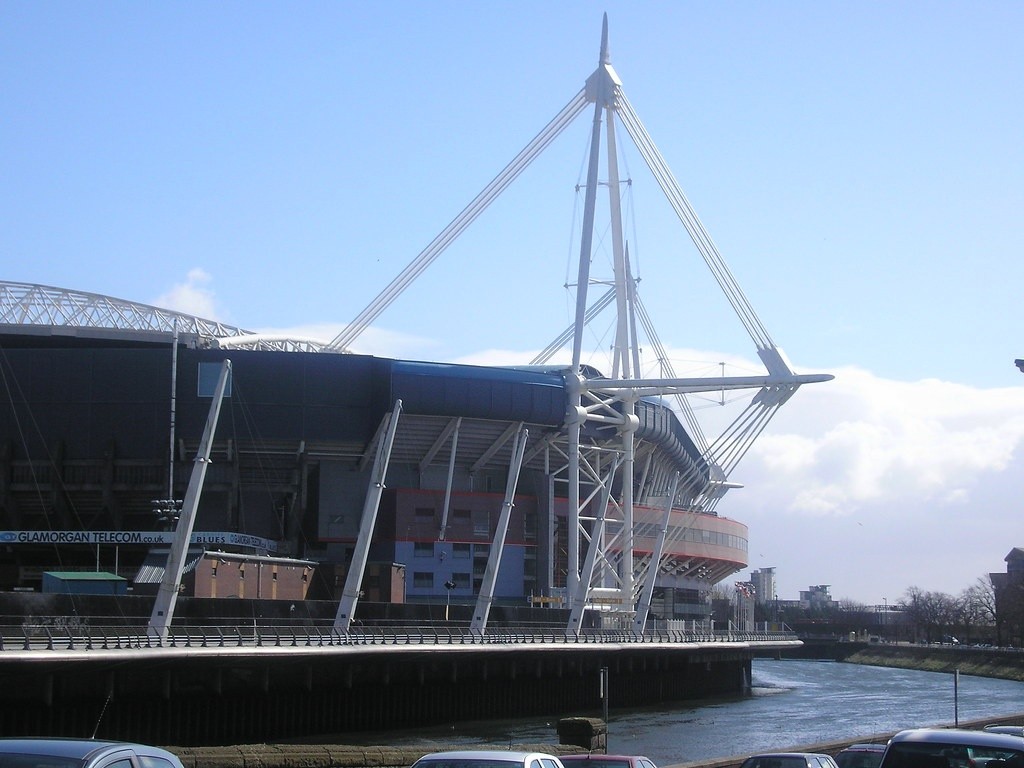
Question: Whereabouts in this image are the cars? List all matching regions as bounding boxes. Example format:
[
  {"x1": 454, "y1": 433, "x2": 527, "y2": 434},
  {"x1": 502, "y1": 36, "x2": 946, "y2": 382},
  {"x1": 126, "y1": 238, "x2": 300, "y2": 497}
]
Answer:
[
  {"x1": 740, "y1": 751, "x2": 839, "y2": 768},
  {"x1": 0, "y1": 738, "x2": 184, "y2": 768},
  {"x1": 408, "y1": 750, "x2": 565, "y2": 768},
  {"x1": 833, "y1": 742, "x2": 887, "y2": 768},
  {"x1": 557, "y1": 753, "x2": 657, "y2": 768}
]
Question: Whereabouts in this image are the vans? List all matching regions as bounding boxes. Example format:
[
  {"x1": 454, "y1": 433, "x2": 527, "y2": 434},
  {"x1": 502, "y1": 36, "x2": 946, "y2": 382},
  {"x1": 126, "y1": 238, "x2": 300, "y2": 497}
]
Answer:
[{"x1": 877, "y1": 723, "x2": 1024, "y2": 768}]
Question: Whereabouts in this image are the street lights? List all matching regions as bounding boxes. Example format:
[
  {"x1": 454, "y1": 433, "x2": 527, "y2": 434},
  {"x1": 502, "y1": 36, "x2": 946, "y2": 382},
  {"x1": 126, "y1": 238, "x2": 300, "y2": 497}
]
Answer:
[{"x1": 882, "y1": 598, "x2": 886, "y2": 640}]
